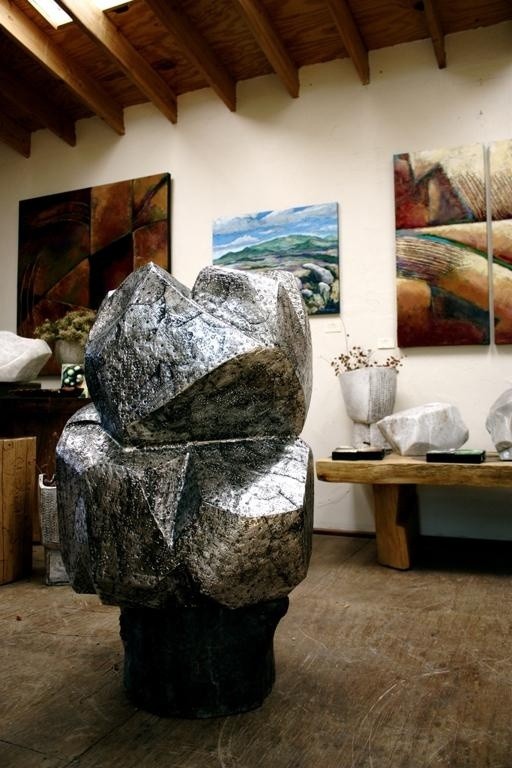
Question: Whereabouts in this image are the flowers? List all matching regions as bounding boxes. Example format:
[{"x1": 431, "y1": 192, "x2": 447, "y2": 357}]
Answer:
[
  {"x1": 330, "y1": 346, "x2": 407, "y2": 376},
  {"x1": 33, "y1": 310, "x2": 98, "y2": 349}
]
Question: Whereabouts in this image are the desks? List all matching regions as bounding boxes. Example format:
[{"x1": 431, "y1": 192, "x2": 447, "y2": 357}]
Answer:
[{"x1": 315, "y1": 450, "x2": 511, "y2": 570}]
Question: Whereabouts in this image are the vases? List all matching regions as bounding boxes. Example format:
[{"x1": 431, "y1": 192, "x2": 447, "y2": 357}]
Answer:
[
  {"x1": 53, "y1": 336, "x2": 85, "y2": 363},
  {"x1": 338, "y1": 368, "x2": 397, "y2": 450}
]
